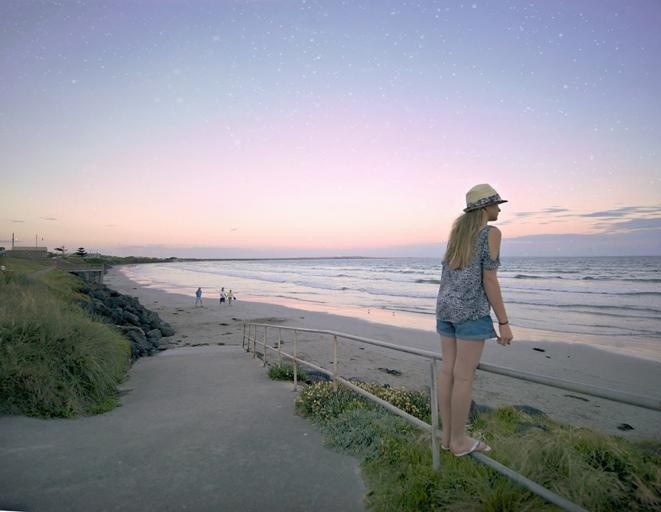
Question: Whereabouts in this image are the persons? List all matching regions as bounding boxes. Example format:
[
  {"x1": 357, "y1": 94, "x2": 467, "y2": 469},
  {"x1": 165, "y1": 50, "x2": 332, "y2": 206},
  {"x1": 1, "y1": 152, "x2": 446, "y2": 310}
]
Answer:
[
  {"x1": 220, "y1": 287, "x2": 229, "y2": 306},
  {"x1": 435, "y1": 182, "x2": 515, "y2": 458},
  {"x1": 195, "y1": 287, "x2": 205, "y2": 308},
  {"x1": 227, "y1": 290, "x2": 235, "y2": 305}
]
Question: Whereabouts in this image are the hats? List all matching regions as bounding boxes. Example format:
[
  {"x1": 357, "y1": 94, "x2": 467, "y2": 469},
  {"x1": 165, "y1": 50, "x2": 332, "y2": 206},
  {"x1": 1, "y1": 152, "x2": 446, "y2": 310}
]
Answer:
[{"x1": 463, "y1": 184, "x2": 508, "y2": 213}]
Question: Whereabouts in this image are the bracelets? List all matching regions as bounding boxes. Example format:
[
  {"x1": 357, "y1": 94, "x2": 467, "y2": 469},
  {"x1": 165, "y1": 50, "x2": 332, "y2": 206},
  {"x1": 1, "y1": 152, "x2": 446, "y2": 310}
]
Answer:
[{"x1": 499, "y1": 321, "x2": 509, "y2": 325}]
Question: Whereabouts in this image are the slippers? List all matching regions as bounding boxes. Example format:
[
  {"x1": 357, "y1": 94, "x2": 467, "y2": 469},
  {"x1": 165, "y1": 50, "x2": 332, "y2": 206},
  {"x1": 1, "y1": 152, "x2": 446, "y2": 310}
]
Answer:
[
  {"x1": 441, "y1": 444, "x2": 450, "y2": 450},
  {"x1": 453, "y1": 439, "x2": 492, "y2": 456}
]
